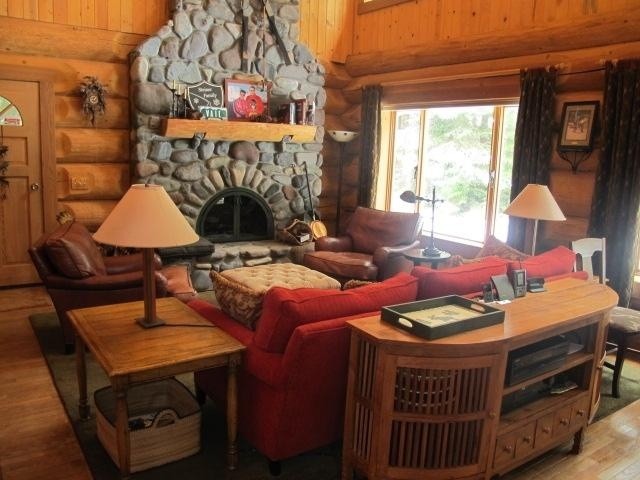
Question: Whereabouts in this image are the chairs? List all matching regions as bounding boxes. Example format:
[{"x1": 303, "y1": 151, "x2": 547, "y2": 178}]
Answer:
[
  {"x1": 29, "y1": 220, "x2": 197, "y2": 355},
  {"x1": 571, "y1": 237, "x2": 640, "y2": 397},
  {"x1": 304, "y1": 206, "x2": 420, "y2": 283}
]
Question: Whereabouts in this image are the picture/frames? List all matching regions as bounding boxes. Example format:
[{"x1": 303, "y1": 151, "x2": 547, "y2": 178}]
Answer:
[
  {"x1": 224, "y1": 78, "x2": 271, "y2": 122},
  {"x1": 556, "y1": 100, "x2": 600, "y2": 149}
]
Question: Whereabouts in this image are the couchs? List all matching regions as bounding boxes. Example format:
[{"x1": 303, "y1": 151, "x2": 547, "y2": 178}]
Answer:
[{"x1": 184, "y1": 235, "x2": 590, "y2": 477}]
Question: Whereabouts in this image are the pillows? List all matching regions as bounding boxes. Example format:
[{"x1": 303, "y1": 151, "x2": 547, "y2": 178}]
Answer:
[{"x1": 210, "y1": 270, "x2": 266, "y2": 331}]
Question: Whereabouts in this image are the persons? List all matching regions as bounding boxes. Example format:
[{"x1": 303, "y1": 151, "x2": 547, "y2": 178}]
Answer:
[
  {"x1": 231, "y1": 89, "x2": 249, "y2": 118},
  {"x1": 244, "y1": 86, "x2": 265, "y2": 117}
]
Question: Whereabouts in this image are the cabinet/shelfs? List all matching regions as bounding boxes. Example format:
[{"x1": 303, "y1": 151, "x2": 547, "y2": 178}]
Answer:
[{"x1": 340, "y1": 277, "x2": 620, "y2": 480}]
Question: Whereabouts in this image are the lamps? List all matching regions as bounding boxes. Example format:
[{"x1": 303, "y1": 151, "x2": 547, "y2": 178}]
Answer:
[
  {"x1": 399, "y1": 186, "x2": 444, "y2": 256},
  {"x1": 93, "y1": 184, "x2": 200, "y2": 328},
  {"x1": 504, "y1": 184, "x2": 567, "y2": 256}
]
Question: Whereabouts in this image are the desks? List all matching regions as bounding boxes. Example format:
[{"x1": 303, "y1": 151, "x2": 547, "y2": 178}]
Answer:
[{"x1": 66, "y1": 297, "x2": 247, "y2": 480}]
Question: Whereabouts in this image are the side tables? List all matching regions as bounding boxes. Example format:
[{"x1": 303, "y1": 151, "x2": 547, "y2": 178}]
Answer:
[{"x1": 405, "y1": 249, "x2": 451, "y2": 269}]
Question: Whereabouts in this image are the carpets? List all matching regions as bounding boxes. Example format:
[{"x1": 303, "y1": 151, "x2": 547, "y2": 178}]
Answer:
[{"x1": 29, "y1": 291, "x2": 640, "y2": 480}]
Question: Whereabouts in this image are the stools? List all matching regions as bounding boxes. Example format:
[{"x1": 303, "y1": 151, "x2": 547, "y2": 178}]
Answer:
[{"x1": 220, "y1": 262, "x2": 341, "y2": 295}]
